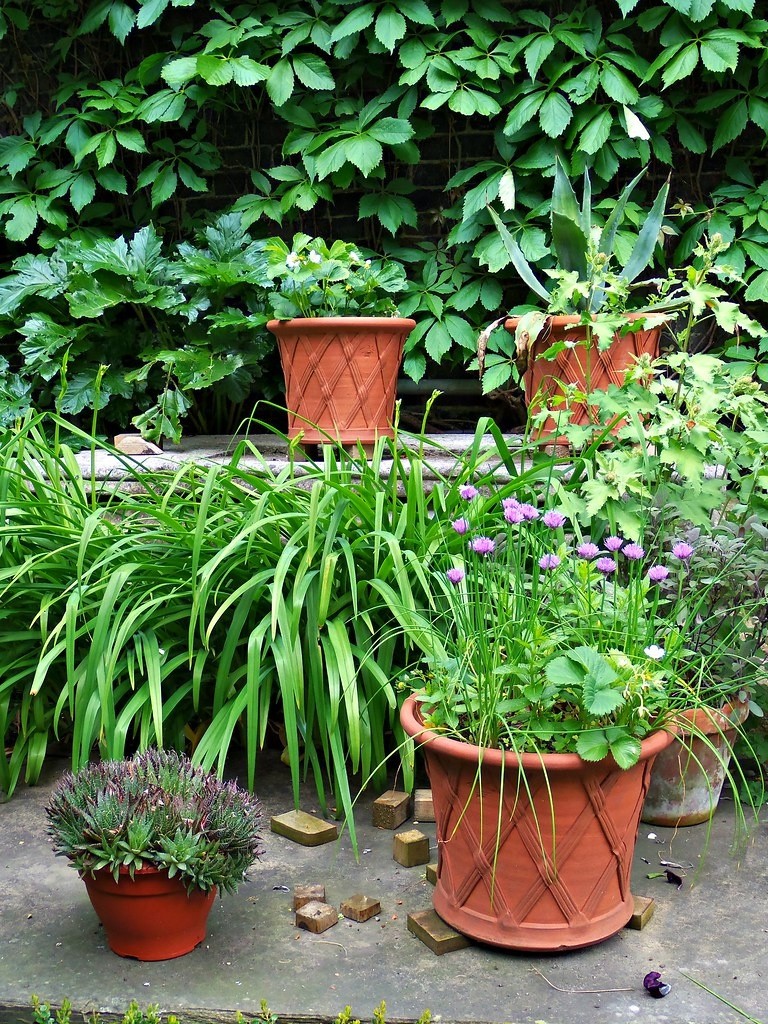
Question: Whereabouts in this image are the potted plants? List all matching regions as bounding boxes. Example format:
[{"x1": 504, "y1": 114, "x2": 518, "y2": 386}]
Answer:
[
  {"x1": 489, "y1": 108, "x2": 690, "y2": 444},
  {"x1": 48, "y1": 748, "x2": 267, "y2": 962}
]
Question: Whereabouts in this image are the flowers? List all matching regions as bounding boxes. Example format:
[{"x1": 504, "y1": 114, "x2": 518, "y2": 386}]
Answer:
[
  {"x1": 555, "y1": 447, "x2": 768, "y2": 702},
  {"x1": 265, "y1": 238, "x2": 410, "y2": 322},
  {"x1": 420, "y1": 463, "x2": 721, "y2": 766}
]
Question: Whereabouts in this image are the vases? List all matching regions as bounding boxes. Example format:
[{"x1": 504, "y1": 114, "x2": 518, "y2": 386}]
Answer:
[
  {"x1": 640, "y1": 683, "x2": 750, "y2": 828},
  {"x1": 401, "y1": 688, "x2": 678, "y2": 951},
  {"x1": 265, "y1": 317, "x2": 416, "y2": 442}
]
